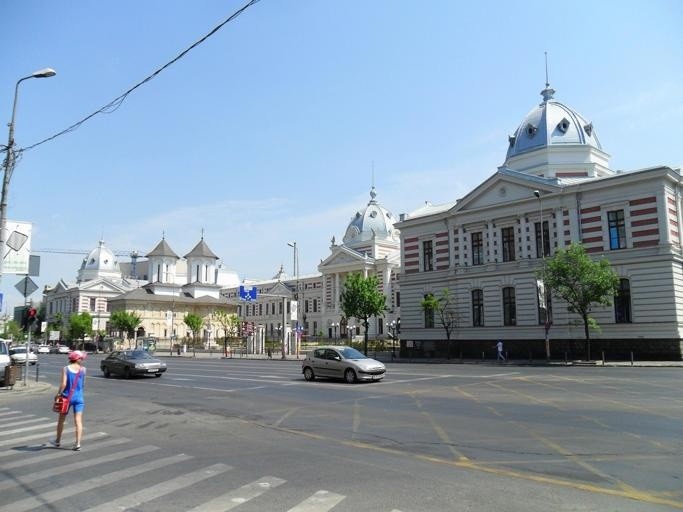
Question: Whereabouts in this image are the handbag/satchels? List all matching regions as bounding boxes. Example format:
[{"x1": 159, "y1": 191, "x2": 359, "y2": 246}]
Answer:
[{"x1": 57, "y1": 392, "x2": 62, "y2": 397}]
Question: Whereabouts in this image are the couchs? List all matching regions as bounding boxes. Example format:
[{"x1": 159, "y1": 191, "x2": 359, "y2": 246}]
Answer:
[{"x1": 27, "y1": 309, "x2": 36, "y2": 324}]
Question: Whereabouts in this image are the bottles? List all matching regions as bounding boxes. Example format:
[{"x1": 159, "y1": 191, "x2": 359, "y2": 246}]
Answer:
[{"x1": 69, "y1": 350, "x2": 87, "y2": 360}]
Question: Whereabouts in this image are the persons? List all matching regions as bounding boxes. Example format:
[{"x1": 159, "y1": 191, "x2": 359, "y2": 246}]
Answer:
[
  {"x1": 50, "y1": 350, "x2": 87, "y2": 451},
  {"x1": 495, "y1": 339, "x2": 505, "y2": 361}
]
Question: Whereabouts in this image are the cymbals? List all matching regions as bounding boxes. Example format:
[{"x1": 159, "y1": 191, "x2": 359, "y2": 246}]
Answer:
[{"x1": 30, "y1": 246, "x2": 147, "y2": 278}]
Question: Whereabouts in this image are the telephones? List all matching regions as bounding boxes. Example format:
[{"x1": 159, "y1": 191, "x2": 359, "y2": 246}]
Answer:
[{"x1": 240, "y1": 287, "x2": 256, "y2": 301}]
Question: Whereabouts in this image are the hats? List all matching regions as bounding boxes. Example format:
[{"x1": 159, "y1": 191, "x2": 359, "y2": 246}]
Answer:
[
  {"x1": 302, "y1": 345, "x2": 385, "y2": 383},
  {"x1": 10, "y1": 347, "x2": 37, "y2": 366},
  {"x1": 6, "y1": 339, "x2": 70, "y2": 355},
  {"x1": 100, "y1": 350, "x2": 168, "y2": 380}
]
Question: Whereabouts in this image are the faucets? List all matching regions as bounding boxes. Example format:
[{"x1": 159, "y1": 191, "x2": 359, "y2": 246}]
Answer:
[
  {"x1": 72, "y1": 446, "x2": 81, "y2": 451},
  {"x1": 50, "y1": 438, "x2": 60, "y2": 447}
]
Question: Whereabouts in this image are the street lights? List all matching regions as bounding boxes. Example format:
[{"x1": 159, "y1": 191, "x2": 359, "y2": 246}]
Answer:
[{"x1": 53, "y1": 396, "x2": 68, "y2": 414}]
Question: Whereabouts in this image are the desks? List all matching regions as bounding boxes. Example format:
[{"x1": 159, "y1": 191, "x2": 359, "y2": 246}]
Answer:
[
  {"x1": 531, "y1": 189, "x2": 554, "y2": 362},
  {"x1": 393, "y1": 319, "x2": 401, "y2": 351},
  {"x1": 386, "y1": 322, "x2": 393, "y2": 335},
  {"x1": 0, "y1": 66, "x2": 57, "y2": 286},
  {"x1": 345, "y1": 324, "x2": 355, "y2": 348},
  {"x1": 287, "y1": 240, "x2": 300, "y2": 355},
  {"x1": 331, "y1": 322, "x2": 340, "y2": 346}
]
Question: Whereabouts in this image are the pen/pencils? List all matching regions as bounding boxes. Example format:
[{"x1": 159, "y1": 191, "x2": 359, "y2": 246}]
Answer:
[{"x1": 0, "y1": 338, "x2": 11, "y2": 382}]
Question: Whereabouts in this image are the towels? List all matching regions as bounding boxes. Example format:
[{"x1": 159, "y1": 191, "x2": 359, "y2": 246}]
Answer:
[
  {"x1": 4, "y1": 365, "x2": 15, "y2": 386},
  {"x1": 180, "y1": 345, "x2": 186, "y2": 352}
]
